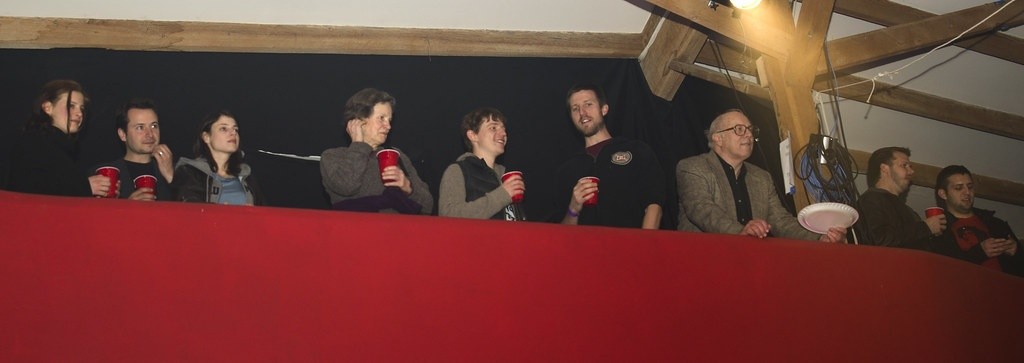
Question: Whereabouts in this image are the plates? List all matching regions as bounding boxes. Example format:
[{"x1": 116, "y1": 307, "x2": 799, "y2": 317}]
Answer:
[{"x1": 797, "y1": 202, "x2": 859, "y2": 235}]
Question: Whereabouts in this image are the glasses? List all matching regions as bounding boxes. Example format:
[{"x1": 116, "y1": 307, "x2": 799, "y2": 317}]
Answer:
[{"x1": 717, "y1": 124, "x2": 760, "y2": 138}]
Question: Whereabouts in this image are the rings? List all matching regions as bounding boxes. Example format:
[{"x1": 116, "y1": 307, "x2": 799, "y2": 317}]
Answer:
[{"x1": 159, "y1": 151, "x2": 164, "y2": 156}]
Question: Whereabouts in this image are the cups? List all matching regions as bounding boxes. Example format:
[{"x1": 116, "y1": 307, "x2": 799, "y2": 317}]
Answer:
[
  {"x1": 134, "y1": 175, "x2": 158, "y2": 195},
  {"x1": 578, "y1": 176, "x2": 600, "y2": 208},
  {"x1": 925, "y1": 207, "x2": 944, "y2": 218},
  {"x1": 501, "y1": 170, "x2": 524, "y2": 203},
  {"x1": 377, "y1": 149, "x2": 400, "y2": 183},
  {"x1": 95, "y1": 166, "x2": 120, "y2": 199}
]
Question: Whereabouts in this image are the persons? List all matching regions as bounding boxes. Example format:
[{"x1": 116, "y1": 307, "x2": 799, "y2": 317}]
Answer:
[
  {"x1": 857, "y1": 147, "x2": 947, "y2": 254},
  {"x1": 676, "y1": 109, "x2": 847, "y2": 242},
  {"x1": 553, "y1": 84, "x2": 670, "y2": 229},
  {"x1": 170, "y1": 111, "x2": 267, "y2": 206},
  {"x1": 320, "y1": 88, "x2": 434, "y2": 214},
  {"x1": 109, "y1": 99, "x2": 176, "y2": 202},
  {"x1": 932, "y1": 165, "x2": 1024, "y2": 276},
  {"x1": 9, "y1": 80, "x2": 122, "y2": 199},
  {"x1": 439, "y1": 108, "x2": 525, "y2": 221}
]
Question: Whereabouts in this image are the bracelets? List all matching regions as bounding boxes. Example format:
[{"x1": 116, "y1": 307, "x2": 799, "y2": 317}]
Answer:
[{"x1": 567, "y1": 206, "x2": 579, "y2": 217}]
